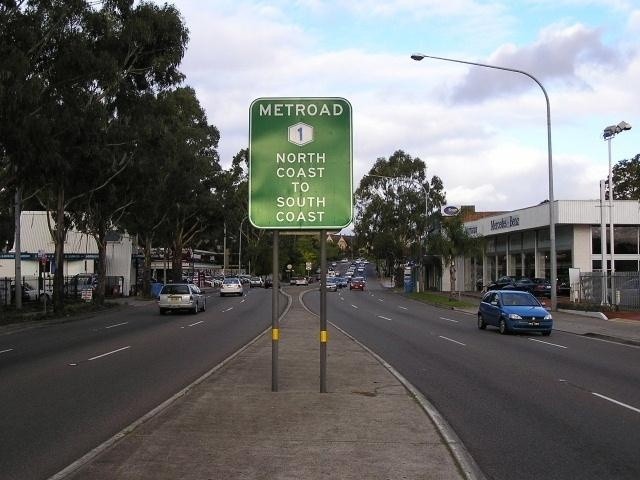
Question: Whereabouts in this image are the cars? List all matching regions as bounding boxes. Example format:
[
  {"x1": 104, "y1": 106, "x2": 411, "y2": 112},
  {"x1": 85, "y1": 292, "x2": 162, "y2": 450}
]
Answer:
[
  {"x1": 9, "y1": 281, "x2": 52, "y2": 302},
  {"x1": 290, "y1": 258, "x2": 370, "y2": 292},
  {"x1": 182, "y1": 269, "x2": 281, "y2": 297},
  {"x1": 608, "y1": 276, "x2": 639, "y2": 308},
  {"x1": 476, "y1": 274, "x2": 570, "y2": 336}
]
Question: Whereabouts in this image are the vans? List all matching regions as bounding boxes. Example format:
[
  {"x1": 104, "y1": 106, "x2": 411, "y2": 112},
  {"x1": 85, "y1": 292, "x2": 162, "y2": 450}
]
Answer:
[{"x1": 156, "y1": 283, "x2": 208, "y2": 316}]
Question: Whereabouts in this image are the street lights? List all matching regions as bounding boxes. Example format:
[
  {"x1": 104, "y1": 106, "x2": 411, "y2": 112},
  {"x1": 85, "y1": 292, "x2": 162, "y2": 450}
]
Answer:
[
  {"x1": 412, "y1": 52, "x2": 560, "y2": 312},
  {"x1": 602, "y1": 120, "x2": 632, "y2": 309},
  {"x1": 363, "y1": 171, "x2": 430, "y2": 253}
]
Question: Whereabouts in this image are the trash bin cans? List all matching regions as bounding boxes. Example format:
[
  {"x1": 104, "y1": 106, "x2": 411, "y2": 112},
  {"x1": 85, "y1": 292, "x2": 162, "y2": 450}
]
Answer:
[{"x1": 111, "y1": 284, "x2": 120, "y2": 298}]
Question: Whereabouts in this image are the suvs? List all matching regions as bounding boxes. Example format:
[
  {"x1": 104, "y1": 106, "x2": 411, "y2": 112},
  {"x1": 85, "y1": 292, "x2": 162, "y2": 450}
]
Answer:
[{"x1": 63, "y1": 271, "x2": 98, "y2": 299}]
